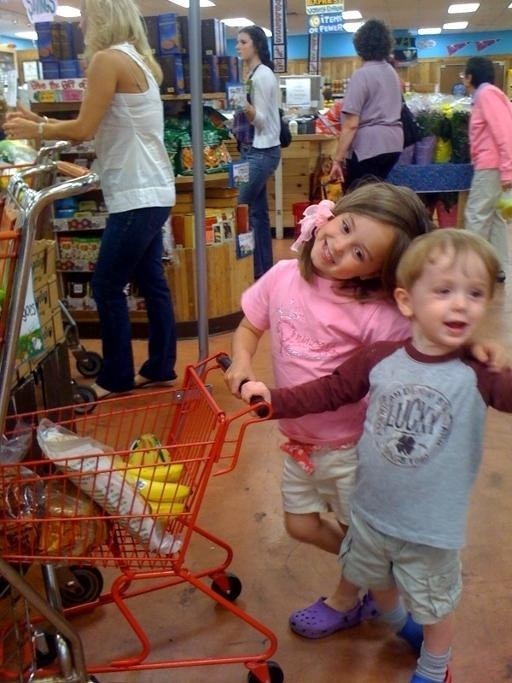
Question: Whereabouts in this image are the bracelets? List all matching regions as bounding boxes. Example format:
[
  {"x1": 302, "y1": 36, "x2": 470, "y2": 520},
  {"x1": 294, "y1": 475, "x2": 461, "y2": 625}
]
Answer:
[
  {"x1": 39, "y1": 122, "x2": 46, "y2": 140},
  {"x1": 43, "y1": 115, "x2": 49, "y2": 123}
]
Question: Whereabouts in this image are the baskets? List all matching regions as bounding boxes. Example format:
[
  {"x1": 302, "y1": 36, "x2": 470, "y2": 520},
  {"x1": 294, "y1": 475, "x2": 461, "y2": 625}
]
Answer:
[{"x1": 292, "y1": 178, "x2": 346, "y2": 241}]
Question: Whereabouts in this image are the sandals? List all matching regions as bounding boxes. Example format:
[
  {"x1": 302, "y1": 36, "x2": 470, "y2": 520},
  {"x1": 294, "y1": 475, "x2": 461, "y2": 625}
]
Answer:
[
  {"x1": 134, "y1": 374, "x2": 178, "y2": 388},
  {"x1": 90, "y1": 383, "x2": 132, "y2": 398},
  {"x1": 497, "y1": 270, "x2": 506, "y2": 283},
  {"x1": 290, "y1": 596, "x2": 381, "y2": 639}
]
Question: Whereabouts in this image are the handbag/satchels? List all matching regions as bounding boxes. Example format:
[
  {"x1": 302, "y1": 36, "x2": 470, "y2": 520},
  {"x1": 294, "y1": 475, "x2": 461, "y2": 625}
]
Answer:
[
  {"x1": 401, "y1": 104, "x2": 424, "y2": 148},
  {"x1": 278, "y1": 109, "x2": 292, "y2": 147}
]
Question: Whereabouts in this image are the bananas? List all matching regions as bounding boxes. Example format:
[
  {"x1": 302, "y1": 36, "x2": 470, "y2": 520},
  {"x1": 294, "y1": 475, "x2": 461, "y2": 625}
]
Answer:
[
  {"x1": 116, "y1": 431, "x2": 192, "y2": 524},
  {"x1": 44, "y1": 505, "x2": 106, "y2": 557}
]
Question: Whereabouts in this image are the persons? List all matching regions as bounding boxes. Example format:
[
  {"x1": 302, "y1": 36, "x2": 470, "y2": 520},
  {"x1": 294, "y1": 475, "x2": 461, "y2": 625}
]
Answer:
[
  {"x1": 233, "y1": 24, "x2": 283, "y2": 278},
  {"x1": 241, "y1": 226, "x2": 512, "y2": 682},
  {"x1": 1, "y1": 0, "x2": 179, "y2": 398},
  {"x1": 327, "y1": 15, "x2": 405, "y2": 192},
  {"x1": 460, "y1": 55, "x2": 512, "y2": 281},
  {"x1": 220, "y1": 180, "x2": 435, "y2": 638}
]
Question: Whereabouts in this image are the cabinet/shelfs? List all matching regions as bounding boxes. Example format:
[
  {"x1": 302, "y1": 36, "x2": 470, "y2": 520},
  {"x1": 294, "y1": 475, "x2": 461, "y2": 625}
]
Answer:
[{"x1": 33, "y1": 92, "x2": 256, "y2": 326}]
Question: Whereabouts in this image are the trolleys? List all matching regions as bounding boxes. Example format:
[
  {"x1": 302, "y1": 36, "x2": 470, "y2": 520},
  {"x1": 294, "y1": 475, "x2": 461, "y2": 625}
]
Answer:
[
  {"x1": 1, "y1": 140, "x2": 103, "y2": 601},
  {"x1": 1, "y1": 352, "x2": 290, "y2": 683}
]
